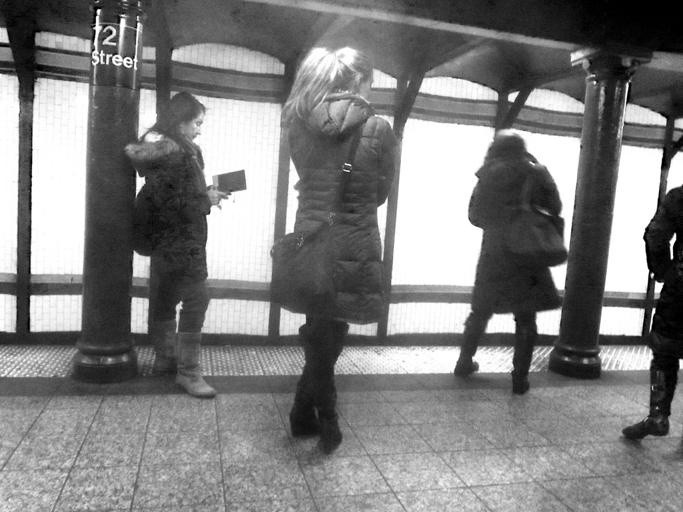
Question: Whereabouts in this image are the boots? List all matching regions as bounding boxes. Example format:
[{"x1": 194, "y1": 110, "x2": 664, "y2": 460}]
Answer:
[
  {"x1": 622, "y1": 359, "x2": 680, "y2": 440},
  {"x1": 149, "y1": 319, "x2": 176, "y2": 377},
  {"x1": 288, "y1": 318, "x2": 349, "y2": 447},
  {"x1": 454, "y1": 312, "x2": 490, "y2": 377},
  {"x1": 510, "y1": 317, "x2": 537, "y2": 394},
  {"x1": 175, "y1": 330, "x2": 218, "y2": 399}
]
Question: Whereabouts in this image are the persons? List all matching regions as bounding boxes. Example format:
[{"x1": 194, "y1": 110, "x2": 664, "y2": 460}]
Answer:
[
  {"x1": 453, "y1": 128, "x2": 562, "y2": 395},
  {"x1": 622, "y1": 185, "x2": 683, "y2": 440},
  {"x1": 272, "y1": 46, "x2": 395, "y2": 454},
  {"x1": 123, "y1": 92, "x2": 229, "y2": 398}
]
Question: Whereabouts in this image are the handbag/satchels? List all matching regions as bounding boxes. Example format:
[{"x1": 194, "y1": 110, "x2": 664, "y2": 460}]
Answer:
[
  {"x1": 269, "y1": 226, "x2": 337, "y2": 315},
  {"x1": 132, "y1": 183, "x2": 163, "y2": 257},
  {"x1": 503, "y1": 206, "x2": 568, "y2": 268}
]
